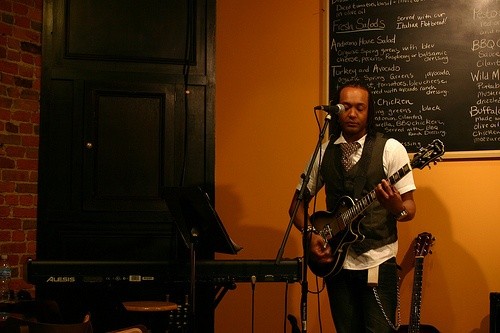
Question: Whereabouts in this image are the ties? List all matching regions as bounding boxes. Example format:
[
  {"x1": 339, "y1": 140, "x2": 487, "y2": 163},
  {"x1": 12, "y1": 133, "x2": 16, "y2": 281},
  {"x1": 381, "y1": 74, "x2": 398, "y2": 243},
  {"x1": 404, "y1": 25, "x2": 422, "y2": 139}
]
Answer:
[{"x1": 338, "y1": 142, "x2": 359, "y2": 169}]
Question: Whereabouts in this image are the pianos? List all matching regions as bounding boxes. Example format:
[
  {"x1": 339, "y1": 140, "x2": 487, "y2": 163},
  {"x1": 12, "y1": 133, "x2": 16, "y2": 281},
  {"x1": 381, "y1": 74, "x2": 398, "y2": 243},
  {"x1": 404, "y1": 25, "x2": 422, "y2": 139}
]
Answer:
[{"x1": 21, "y1": 255, "x2": 308, "y2": 333}]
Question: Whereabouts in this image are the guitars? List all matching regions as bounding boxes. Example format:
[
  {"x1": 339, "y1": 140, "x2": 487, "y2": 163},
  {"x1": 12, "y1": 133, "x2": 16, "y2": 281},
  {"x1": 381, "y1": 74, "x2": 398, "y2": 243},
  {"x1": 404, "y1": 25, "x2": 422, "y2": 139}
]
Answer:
[
  {"x1": 303, "y1": 138, "x2": 445, "y2": 278},
  {"x1": 386, "y1": 231, "x2": 443, "y2": 333}
]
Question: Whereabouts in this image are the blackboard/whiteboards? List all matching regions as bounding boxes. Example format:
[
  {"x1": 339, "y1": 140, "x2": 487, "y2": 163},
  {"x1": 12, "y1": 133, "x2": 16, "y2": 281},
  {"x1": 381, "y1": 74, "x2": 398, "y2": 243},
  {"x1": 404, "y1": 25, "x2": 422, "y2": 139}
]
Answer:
[{"x1": 322, "y1": 0, "x2": 496, "y2": 159}]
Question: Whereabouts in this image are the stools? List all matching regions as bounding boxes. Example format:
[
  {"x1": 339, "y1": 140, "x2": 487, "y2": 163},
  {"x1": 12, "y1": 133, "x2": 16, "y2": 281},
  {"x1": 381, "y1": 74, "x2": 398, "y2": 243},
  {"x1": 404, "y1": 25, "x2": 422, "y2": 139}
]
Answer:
[{"x1": 122, "y1": 301, "x2": 178, "y2": 312}]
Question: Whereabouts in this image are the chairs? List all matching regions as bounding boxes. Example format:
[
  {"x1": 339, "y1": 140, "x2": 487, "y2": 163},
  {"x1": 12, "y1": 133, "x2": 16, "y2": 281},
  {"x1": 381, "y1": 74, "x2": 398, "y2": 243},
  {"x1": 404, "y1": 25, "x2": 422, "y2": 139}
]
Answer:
[{"x1": 0, "y1": 300, "x2": 94, "y2": 333}]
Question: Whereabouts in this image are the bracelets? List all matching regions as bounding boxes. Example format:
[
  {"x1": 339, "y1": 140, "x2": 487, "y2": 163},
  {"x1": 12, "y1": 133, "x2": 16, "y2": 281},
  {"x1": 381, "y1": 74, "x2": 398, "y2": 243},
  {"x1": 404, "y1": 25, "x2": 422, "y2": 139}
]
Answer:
[
  {"x1": 392, "y1": 207, "x2": 408, "y2": 220},
  {"x1": 300, "y1": 225, "x2": 317, "y2": 235}
]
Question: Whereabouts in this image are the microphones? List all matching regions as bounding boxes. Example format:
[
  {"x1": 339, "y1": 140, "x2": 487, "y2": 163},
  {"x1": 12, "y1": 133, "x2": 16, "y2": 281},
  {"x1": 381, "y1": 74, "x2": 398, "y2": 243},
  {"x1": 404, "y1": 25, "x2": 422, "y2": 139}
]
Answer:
[{"x1": 315, "y1": 104, "x2": 346, "y2": 113}]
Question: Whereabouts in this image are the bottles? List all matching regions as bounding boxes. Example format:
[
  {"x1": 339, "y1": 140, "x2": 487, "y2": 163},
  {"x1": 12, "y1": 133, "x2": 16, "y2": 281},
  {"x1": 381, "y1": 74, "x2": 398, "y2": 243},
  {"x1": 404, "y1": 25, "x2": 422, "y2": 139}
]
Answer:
[{"x1": 0, "y1": 255, "x2": 11, "y2": 302}]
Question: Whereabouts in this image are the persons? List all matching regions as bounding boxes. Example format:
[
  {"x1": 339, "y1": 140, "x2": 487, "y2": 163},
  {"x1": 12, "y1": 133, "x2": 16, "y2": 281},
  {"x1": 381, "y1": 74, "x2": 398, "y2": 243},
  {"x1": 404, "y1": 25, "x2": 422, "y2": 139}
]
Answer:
[{"x1": 289, "y1": 81, "x2": 416, "y2": 333}]
